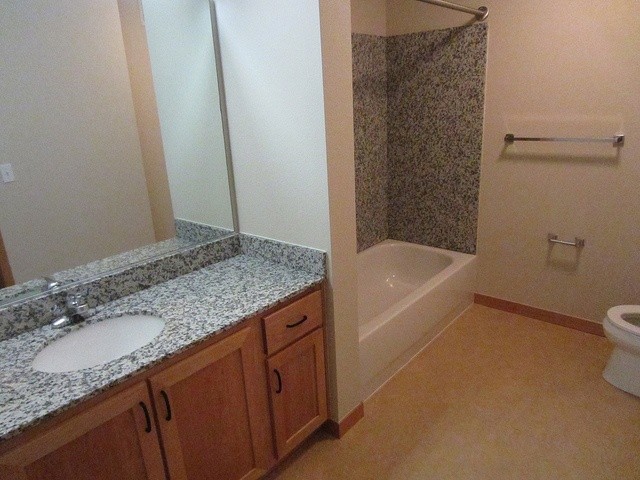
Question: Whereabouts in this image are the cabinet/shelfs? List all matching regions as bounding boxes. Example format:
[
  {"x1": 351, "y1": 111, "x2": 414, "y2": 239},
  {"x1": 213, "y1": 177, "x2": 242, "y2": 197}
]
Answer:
[
  {"x1": 1, "y1": 319, "x2": 270, "y2": 480},
  {"x1": 262, "y1": 290, "x2": 326, "y2": 461}
]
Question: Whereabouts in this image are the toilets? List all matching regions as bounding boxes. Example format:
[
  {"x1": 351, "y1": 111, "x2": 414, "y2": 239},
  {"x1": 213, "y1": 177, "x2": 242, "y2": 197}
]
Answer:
[{"x1": 603, "y1": 304, "x2": 640, "y2": 401}]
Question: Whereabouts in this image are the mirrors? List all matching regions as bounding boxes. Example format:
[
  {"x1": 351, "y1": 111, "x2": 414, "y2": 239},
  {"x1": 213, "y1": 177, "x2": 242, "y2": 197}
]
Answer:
[{"x1": 1, "y1": 0, "x2": 235, "y2": 310}]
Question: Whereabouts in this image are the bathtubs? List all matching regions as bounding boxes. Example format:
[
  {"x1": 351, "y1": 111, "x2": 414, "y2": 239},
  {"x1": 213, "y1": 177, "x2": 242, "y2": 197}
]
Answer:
[{"x1": 357, "y1": 239, "x2": 453, "y2": 347}]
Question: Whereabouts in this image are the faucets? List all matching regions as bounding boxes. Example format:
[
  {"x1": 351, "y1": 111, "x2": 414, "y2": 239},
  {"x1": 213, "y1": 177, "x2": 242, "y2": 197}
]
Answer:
[
  {"x1": 49, "y1": 287, "x2": 89, "y2": 324},
  {"x1": 42, "y1": 272, "x2": 60, "y2": 290}
]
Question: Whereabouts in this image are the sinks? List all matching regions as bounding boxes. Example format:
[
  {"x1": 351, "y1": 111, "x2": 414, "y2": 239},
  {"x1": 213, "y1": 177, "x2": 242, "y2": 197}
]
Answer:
[{"x1": 28, "y1": 307, "x2": 167, "y2": 373}]
